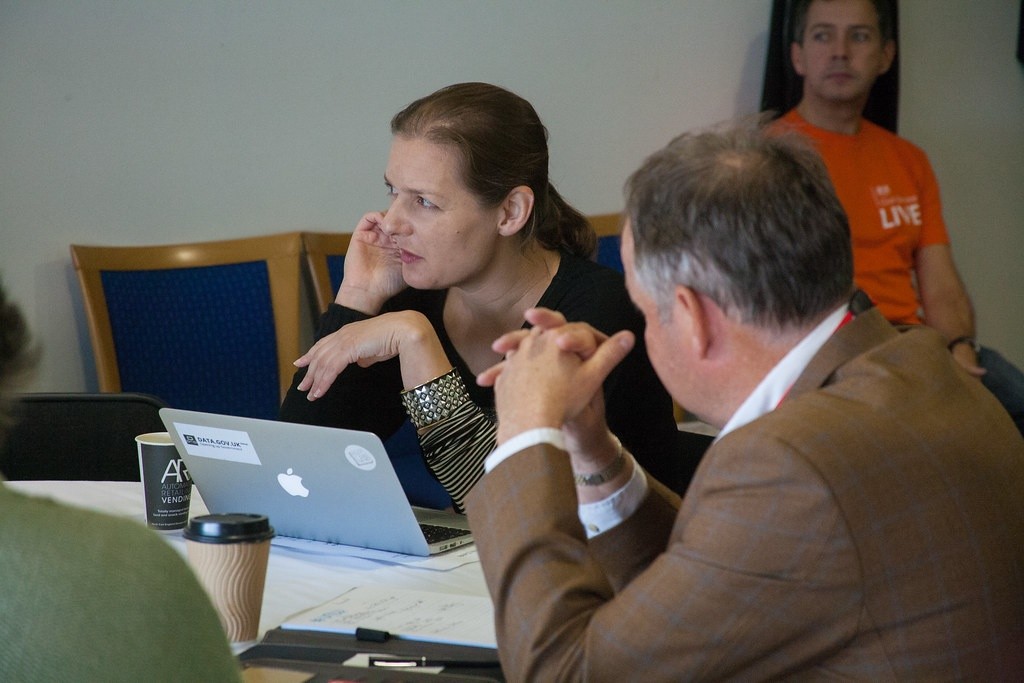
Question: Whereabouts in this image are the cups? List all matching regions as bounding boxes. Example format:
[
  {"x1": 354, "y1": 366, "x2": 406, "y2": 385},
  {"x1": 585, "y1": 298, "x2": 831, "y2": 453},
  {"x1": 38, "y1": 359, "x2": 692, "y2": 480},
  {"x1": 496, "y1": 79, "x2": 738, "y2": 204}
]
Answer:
[
  {"x1": 135, "y1": 432, "x2": 193, "y2": 534},
  {"x1": 182, "y1": 512, "x2": 276, "y2": 649}
]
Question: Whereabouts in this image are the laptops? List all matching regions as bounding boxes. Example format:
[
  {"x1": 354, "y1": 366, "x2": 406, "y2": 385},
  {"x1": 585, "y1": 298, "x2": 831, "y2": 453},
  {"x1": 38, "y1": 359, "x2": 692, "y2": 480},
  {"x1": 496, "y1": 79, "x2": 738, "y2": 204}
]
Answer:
[{"x1": 159, "y1": 406, "x2": 475, "y2": 555}]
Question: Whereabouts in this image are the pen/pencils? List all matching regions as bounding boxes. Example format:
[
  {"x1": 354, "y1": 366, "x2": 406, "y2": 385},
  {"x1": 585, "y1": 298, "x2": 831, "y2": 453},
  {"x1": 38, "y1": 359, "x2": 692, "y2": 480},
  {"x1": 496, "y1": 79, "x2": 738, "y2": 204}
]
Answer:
[{"x1": 367, "y1": 657, "x2": 500, "y2": 668}]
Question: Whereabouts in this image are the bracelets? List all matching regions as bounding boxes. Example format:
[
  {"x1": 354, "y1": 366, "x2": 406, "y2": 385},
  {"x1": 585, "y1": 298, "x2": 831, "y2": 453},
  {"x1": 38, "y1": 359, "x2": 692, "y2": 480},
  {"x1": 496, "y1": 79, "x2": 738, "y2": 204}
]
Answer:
[
  {"x1": 398, "y1": 367, "x2": 470, "y2": 429},
  {"x1": 575, "y1": 435, "x2": 625, "y2": 486},
  {"x1": 948, "y1": 336, "x2": 980, "y2": 353}
]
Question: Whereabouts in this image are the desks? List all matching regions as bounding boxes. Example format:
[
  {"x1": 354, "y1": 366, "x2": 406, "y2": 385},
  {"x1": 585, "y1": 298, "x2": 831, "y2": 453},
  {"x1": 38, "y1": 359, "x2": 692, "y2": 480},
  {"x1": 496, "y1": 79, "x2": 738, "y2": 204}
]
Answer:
[{"x1": 1, "y1": 476, "x2": 498, "y2": 657}]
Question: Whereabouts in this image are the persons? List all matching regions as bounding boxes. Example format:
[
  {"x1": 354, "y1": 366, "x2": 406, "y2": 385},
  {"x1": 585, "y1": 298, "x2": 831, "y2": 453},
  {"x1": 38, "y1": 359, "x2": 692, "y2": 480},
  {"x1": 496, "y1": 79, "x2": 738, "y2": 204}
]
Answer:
[
  {"x1": 0, "y1": 292, "x2": 251, "y2": 683},
  {"x1": 275, "y1": 82, "x2": 683, "y2": 519},
  {"x1": 465, "y1": 122, "x2": 1023, "y2": 683},
  {"x1": 763, "y1": 0, "x2": 1024, "y2": 430}
]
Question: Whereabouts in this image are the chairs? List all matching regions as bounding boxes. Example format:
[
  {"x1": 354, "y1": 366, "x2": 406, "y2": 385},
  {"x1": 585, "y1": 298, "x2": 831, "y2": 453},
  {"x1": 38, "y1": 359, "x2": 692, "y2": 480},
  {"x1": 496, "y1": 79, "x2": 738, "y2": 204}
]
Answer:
[
  {"x1": 577, "y1": 209, "x2": 686, "y2": 425},
  {"x1": 303, "y1": 231, "x2": 356, "y2": 318},
  {"x1": 0, "y1": 390, "x2": 171, "y2": 481},
  {"x1": 65, "y1": 231, "x2": 304, "y2": 421}
]
